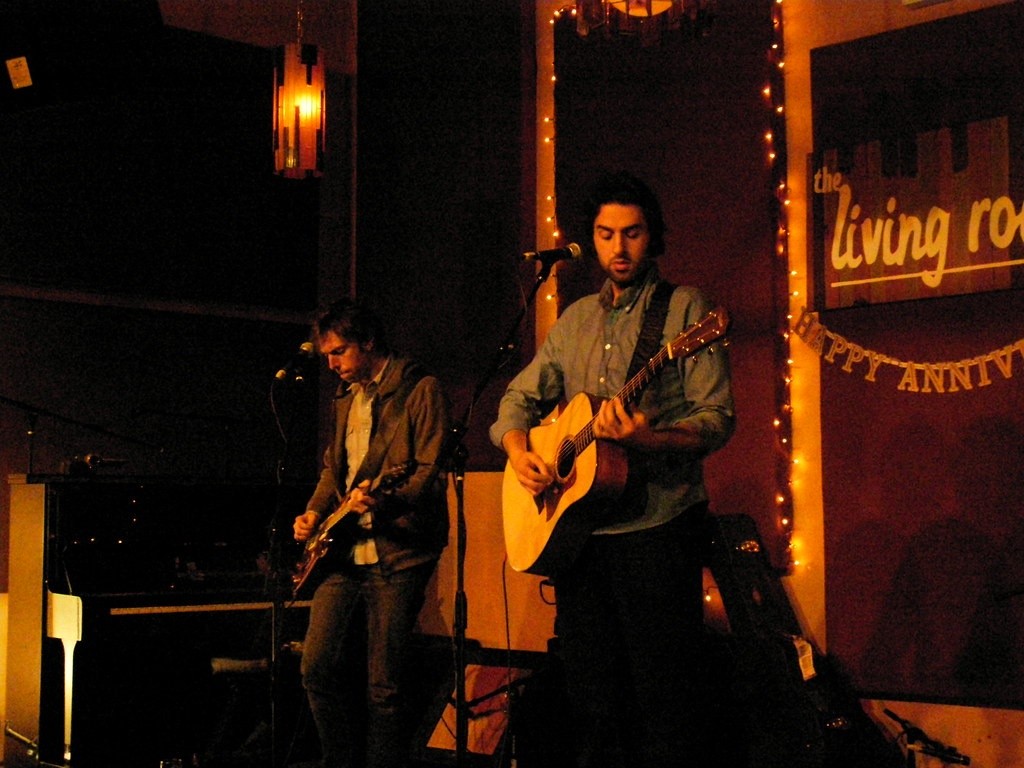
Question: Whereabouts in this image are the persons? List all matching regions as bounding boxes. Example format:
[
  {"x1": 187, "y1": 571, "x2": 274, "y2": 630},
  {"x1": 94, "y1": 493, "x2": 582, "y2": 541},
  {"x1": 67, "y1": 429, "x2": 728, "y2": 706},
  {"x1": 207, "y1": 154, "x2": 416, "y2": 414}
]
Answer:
[
  {"x1": 169, "y1": 556, "x2": 204, "y2": 588},
  {"x1": 252, "y1": 560, "x2": 282, "y2": 597},
  {"x1": 292, "y1": 311, "x2": 450, "y2": 768},
  {"x1": 486, "y1": 179, "x2": 735, "y2": 768}
]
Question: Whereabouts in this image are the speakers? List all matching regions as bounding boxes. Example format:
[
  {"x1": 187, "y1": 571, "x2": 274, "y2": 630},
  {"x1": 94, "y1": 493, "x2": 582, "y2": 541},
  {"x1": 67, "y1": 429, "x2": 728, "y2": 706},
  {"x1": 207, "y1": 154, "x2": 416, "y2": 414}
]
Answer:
[{"x1": 426, "y1": 664, "x2": 535, "y2": 768}]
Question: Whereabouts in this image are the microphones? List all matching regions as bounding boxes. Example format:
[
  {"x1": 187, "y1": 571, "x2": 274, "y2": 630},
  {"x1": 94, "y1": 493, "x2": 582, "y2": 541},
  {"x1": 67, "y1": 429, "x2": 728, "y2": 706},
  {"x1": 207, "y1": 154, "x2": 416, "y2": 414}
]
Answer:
[
  {"x1": 520, "y1": 242, "x2": 583, "y2": 264},
  {"x1": 273, "y1": 342, "x2": 315, "y2": 380},
  {"x1": 906, "y1": 742, "x2": 970, "y2": 765}
]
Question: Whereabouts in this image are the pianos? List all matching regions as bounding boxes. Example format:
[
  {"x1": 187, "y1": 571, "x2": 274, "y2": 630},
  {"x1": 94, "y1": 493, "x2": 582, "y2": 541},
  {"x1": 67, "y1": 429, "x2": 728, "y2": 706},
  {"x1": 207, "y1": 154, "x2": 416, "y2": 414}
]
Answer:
[{"x1": 5, "y1": 474, "x2": 314, "y2": 768}]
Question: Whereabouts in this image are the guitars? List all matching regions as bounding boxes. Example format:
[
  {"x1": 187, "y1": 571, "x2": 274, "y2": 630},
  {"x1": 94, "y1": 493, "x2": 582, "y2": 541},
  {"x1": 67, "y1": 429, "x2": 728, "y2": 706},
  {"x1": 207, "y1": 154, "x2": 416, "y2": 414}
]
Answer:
[
  {"x1": 501, "y1": 304, "x2": 733, "y2": 578},
  {"x1": 289, "y1": 459, "x2": 418, "y2": 595}
]
